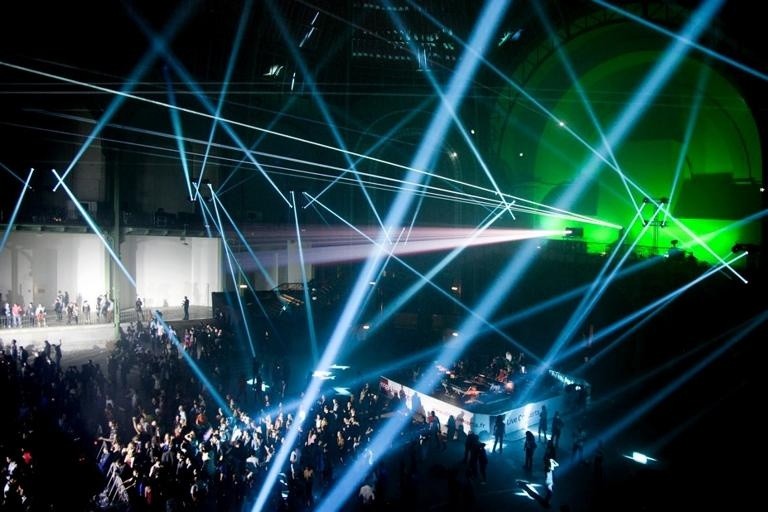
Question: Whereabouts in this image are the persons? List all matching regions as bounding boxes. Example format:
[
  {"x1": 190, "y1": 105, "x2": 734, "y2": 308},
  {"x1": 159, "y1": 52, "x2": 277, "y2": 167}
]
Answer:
[
  {"x1": 493, "y1": 415, "x2": 505, "y2": 454},
  {"x1": 538, "y1": 406, "x2": 547, "y2": 443},
  {"x1": 551, "y1": 411, "x2": 563, "y2": 447},
  {"x1": 523, "y1": 431, "x2": 536, "y2": 470},
  {"x1": 571, "y1": 426, "x2": 586, "y2": 461},
  {"x1": 0, "y1": 291, "x2": 489, "y2": 511},
  {"x1": 544, "y1": 446, "x2": 559, "y2": 507}
]
